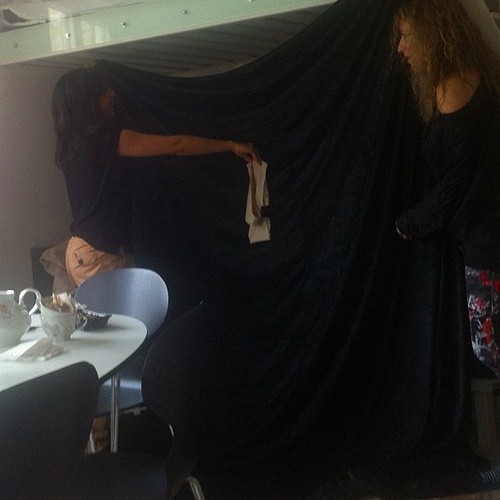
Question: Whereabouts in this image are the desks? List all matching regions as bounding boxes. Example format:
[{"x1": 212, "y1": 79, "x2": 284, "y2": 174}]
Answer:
[{"x1": 0, "y1": 309, "x2": 148, "y2": 454}]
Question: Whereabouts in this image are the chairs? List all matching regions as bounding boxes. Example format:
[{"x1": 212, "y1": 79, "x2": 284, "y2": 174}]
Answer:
[
  {"x1": 73, "y1": 267, "x2": 170, "y2": 454},
  {"x1": 89, "y1": 301, "x2": 219, "y2": 500},
  {"x1": 0, "y1": 360, "x2": 102, "y2": 500}
]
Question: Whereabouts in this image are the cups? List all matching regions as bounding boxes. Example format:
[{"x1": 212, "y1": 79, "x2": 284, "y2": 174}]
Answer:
[{"x1": 36, "y1": 297, "x2": 89, "y2": 343}]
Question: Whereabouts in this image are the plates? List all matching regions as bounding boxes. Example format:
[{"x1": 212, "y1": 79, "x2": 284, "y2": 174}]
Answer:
[{"x1": 75, "y1": 312, "x2": 112, "y2": 332}]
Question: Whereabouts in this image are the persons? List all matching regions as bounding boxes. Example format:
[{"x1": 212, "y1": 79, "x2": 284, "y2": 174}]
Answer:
[
  {"x1": 51, "y1": 67, "x2": 264, "y2": 500},
  {"x1": 391, "y1": 1, "x2": 500, "y2": 500}
]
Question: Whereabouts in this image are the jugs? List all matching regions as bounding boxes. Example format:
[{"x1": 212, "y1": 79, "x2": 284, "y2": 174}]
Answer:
[{"x1": 0, "y1": 288, "x2": 42, "y2": 346}]
